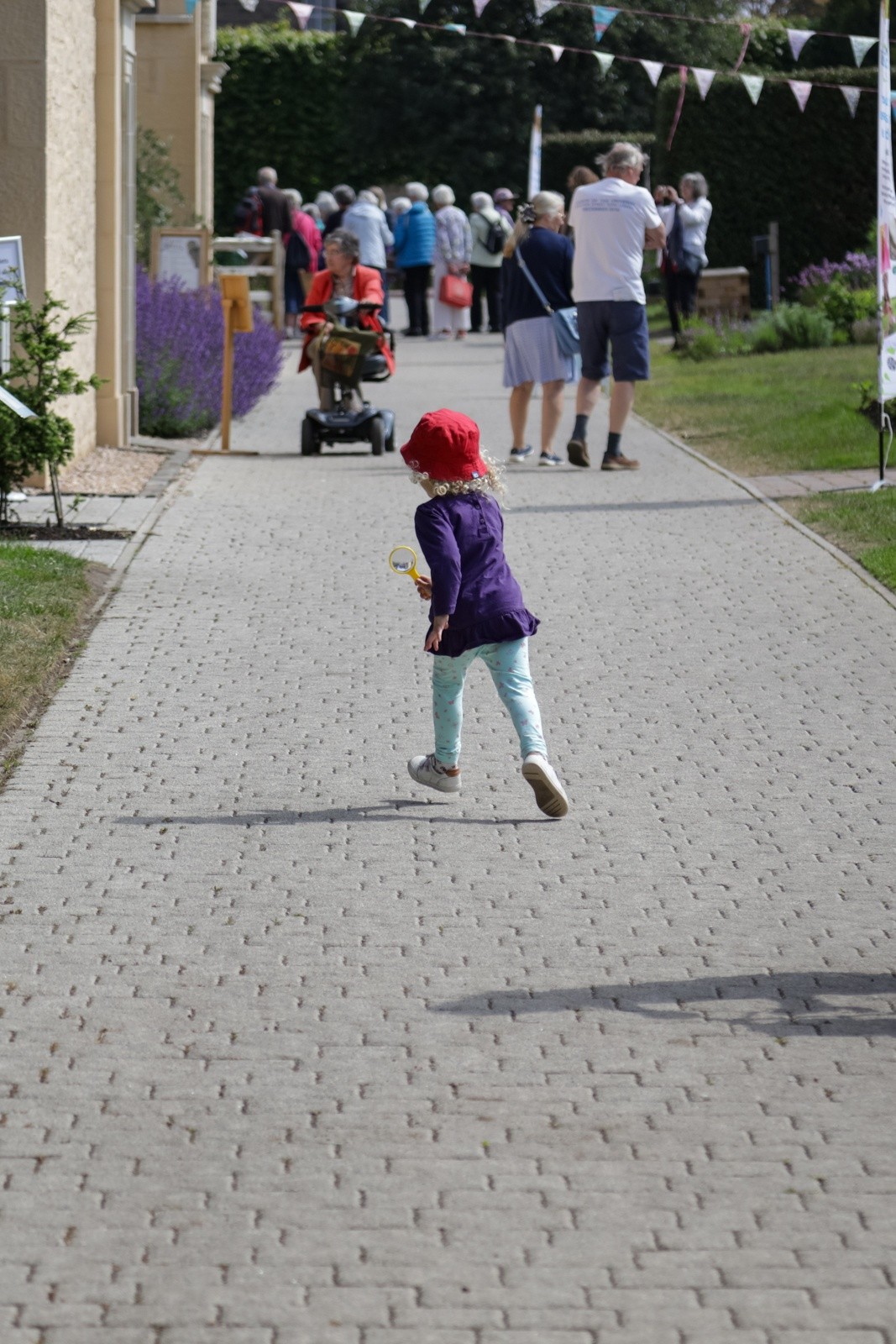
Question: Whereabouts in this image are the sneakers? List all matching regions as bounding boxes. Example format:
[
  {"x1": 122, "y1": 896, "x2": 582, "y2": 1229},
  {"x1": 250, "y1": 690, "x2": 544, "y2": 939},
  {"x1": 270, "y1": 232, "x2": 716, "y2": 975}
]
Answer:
[
  {"x1": 539, "y1": 452, "x2": 566, "y2": 465},
  {"x1": 521, "y1": 754, "x2": 571, "y2": 819},
  {"x1": 600, "y1": 452, "x2": 640, "y2": 469},
  {"x1": 410, "y1": 756, "x2": 464, "y2": 793},
  {"x1": 567, "y1": 440, "x2": 591, "y2": 467},
  {"x1": 509, "y1": 445, "x2": 533, "y2": 462}
]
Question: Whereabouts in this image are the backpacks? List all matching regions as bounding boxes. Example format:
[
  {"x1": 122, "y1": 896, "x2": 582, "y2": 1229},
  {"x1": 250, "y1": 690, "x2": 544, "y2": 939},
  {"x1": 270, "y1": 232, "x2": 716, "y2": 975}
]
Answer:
[
  {"x1": 477, "y1": 213, "x2": 503, "y2": 254},
  {"x1": 284, "y1": 230, "x2": 311, "y2": 281}
]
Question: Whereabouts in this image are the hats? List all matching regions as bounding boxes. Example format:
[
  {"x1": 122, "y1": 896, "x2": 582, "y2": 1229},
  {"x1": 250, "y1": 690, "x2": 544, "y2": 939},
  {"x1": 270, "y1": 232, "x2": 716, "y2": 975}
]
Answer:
[
  {"x1": 495, "y1": 189, "x2": 518, "y2": 200},
  {"x1": 401, "y1": 409, "x2": 488, "y2": 482}
]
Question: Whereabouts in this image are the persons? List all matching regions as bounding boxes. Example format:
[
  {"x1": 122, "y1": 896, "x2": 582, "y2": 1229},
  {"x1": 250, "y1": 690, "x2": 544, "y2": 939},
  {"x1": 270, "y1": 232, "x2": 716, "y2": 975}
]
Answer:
[
  {"x1": 554, "y1": 167, "x2": 599, "y2": 239},
  {"x1": 408, "y1": 408, "x2": 572, "y2": 818},
  {"x1": 653, "y1": 173, "x2": 714, "y2": 355},
  {"x1": 567, "y1": 142, "x2": 666, "y2": 469},
  {"x1": 497, "y1": 189, "x2": 574, "y2": 467},
  {"x1": 241, "y1": 165, "x2": 516, "y2": 341},
  {"x1": 299, "y1": 226, "x2": 388, "y2": 413}
]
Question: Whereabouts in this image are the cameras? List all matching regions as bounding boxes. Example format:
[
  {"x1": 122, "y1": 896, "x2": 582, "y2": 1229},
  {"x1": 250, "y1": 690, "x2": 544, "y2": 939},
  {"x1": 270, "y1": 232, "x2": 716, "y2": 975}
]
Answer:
[{"x1": 661, "y1": 187, "x2": 669, "y2": 196}]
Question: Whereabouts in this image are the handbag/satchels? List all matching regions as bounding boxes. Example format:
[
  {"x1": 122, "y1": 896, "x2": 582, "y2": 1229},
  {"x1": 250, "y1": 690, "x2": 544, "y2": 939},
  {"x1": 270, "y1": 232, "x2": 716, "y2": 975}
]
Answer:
[
  {"x1": 553, "y1": 306, "x2": 581, "y2": 355},
  {"x1": 440, "y1": 272, "x2": 473, "y2": 309}
]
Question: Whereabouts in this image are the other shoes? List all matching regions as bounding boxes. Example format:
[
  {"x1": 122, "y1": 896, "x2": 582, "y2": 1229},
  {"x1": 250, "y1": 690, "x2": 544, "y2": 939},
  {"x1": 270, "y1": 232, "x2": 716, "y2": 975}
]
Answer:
[{"x1": 400, "y1": 326, "x2": 427, "y2": 337}]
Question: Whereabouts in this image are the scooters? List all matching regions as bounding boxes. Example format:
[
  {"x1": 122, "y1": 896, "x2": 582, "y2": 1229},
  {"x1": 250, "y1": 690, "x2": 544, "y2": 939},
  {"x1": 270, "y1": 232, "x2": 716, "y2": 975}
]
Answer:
[{"x1": 294, "y1": 295, "x2": 398, "y2": 459}]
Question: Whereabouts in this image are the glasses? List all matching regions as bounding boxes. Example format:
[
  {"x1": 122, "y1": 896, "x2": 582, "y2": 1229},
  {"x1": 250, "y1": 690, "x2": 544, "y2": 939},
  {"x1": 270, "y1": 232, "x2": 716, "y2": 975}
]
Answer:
[{"x1": 322, "y1": 249, "x2": 341, "y2": 258}]
